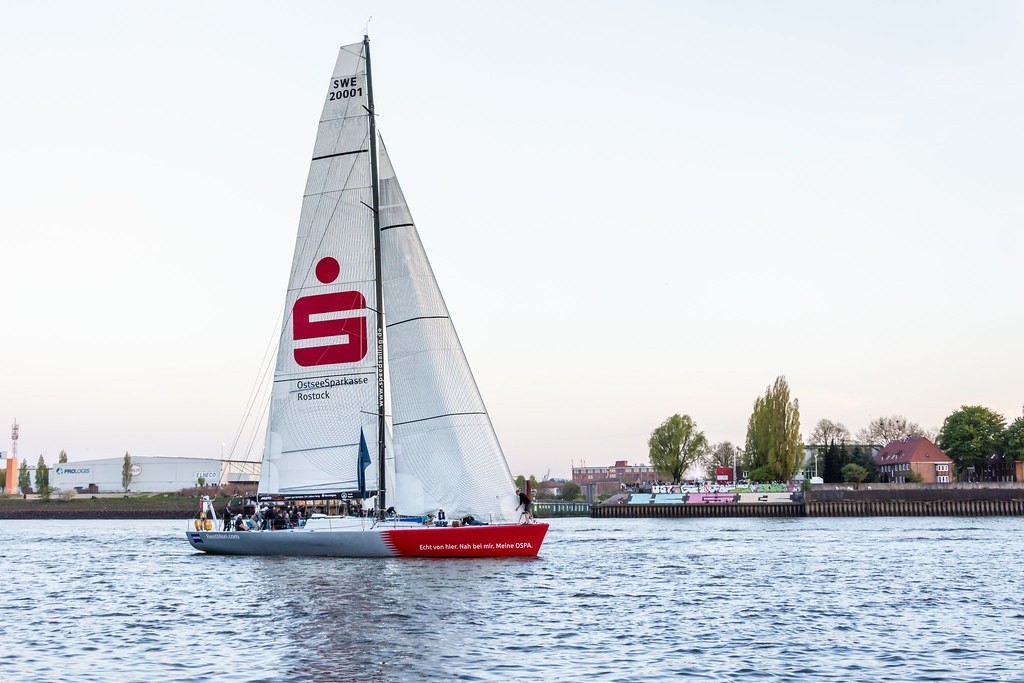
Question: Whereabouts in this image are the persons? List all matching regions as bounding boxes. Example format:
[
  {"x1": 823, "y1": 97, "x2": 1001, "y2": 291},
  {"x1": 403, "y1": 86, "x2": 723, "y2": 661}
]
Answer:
[
  {"x1": 618, "y1": 478, "x2": 798, "y2": 494},
  {"x1": 515, "y1": 490, "x2": 532, "y2": 525},
  {"x1": 436, "y1": 509, "x2": 447, "y2": 527},
  {"x1": 460, "y1": 515, "x2": 489, "y2": 526},
  {"x1": 422, "y1": 514, "x2": 435, "y2": 525},
  {"x1": 222, "y1": 497, "x2": 396, "y2": 531}
]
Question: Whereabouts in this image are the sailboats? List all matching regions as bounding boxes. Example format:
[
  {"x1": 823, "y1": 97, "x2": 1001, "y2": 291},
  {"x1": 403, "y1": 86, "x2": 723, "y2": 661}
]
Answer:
[{"x1": 183, "y1": 33, "x2": 551, "y2": 558}]
